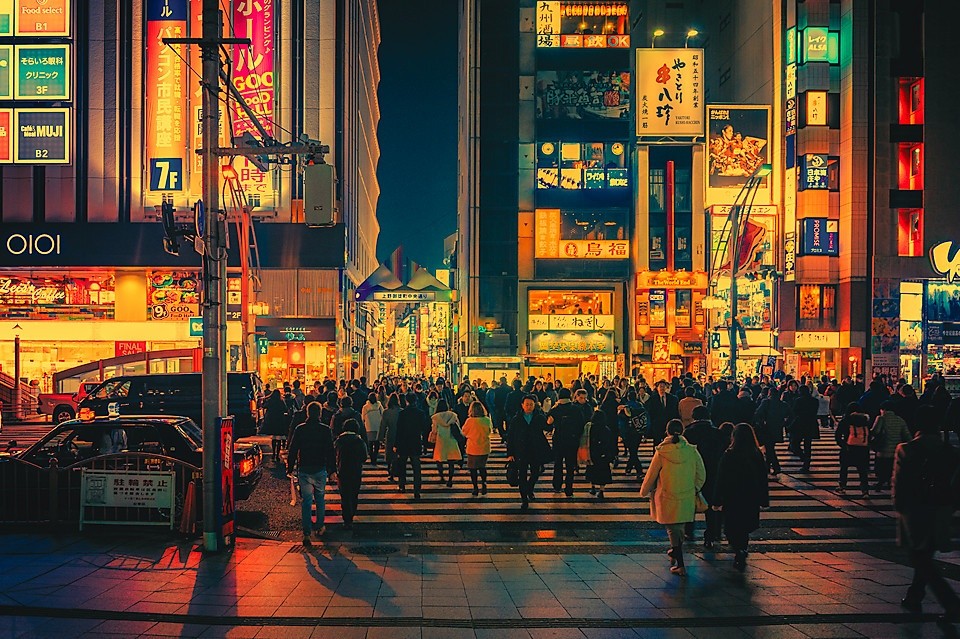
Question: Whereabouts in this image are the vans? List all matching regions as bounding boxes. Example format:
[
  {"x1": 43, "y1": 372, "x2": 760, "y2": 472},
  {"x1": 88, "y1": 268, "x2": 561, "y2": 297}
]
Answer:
[{"x1": 73, "y1": 370, "x2": 264, "y2": 437}]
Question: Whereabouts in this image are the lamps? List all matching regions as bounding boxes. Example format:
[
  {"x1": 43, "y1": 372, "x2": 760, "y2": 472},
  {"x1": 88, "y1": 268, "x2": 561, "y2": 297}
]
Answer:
[
  {"x1": 685, "y1": 29, "x2": 698, "y2": 48},
  {"x1": 652, "y1": 28, "x2": 665, "y2": 48}
]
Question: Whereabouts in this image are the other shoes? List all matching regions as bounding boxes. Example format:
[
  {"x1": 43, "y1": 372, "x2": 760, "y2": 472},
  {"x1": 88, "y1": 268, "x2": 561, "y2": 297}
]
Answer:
[
  {"x1": 589, "y1": 489, "x2": 596, "y2": 495},
  {"x1": 303, "y1": 536, "x2": 312, "y2": 545},
  {"x1": 901, "y1": 586, "x2": 926, "y2": 613},
  {"x1": 937, "y1": 600, "x2": 960, "y2": 631},
  {"x1": 316, "y1": 526, "x2": 326, "y2": 536},
  {"x1": 597, "y1": 493, "x2": 604, "y2": 498}
]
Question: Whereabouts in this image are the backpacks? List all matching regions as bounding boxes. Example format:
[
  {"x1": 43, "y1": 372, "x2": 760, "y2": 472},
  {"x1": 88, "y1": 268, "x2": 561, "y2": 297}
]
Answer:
[{"x1": 846, "y1": 425, "x2": 869, "y2": 446}]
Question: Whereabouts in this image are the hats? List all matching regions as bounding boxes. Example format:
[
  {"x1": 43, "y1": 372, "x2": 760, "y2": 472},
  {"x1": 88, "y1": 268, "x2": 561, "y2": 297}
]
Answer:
[{"x1": 436, "y1": 379, "x2": 444, "y2": 385}]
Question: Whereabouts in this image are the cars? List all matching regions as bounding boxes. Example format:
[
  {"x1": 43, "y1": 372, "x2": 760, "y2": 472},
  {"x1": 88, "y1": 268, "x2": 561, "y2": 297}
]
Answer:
[{"x1": 0, "y1": 402, "x2": 263, "y2": 521}]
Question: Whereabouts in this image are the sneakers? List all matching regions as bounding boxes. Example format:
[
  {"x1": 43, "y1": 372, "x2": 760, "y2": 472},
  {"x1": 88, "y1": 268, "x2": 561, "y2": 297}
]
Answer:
[
  {"x1": 834, "y1": 487, "x2": 846, "y2": 494},
  {"x1": 669, "y1": 565, "x2": 686, "y2": 576},
  {"x1": 862, "y1": 491, "x2": 869, "y2": 498}
]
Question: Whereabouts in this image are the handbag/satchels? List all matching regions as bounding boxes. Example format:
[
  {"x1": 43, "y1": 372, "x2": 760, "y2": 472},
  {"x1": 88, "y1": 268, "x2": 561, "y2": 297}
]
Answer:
[
  {"x1": 870, "y1": 426, "x2": 889, "y2": 447},
  {"x1": 695, "y1": 492, "x2": 708, "y2": 513},
  {"x1": 289, "y1": 477, "x2": 297, "y2": 507},
  {"x1": 505, "y1": 459, "x2": 519, "y2": 487},
  {"x1": 449, "y1": 422, "x2": 464, "y2": 443},
  {"x1": 577, "y1": 447, "x2": 594, "y2": 465}
]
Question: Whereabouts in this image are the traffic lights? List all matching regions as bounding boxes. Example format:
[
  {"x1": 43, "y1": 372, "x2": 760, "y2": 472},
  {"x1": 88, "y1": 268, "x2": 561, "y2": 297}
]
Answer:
[{"x1": 743, "y1": 268, "x2": 768, "y2": 282}]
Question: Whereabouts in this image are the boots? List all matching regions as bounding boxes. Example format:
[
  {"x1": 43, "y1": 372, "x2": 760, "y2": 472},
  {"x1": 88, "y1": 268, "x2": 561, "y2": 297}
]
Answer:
[
  {"x1": 276, "y1": 440, "x2": 281, "y2": 461},
  {"x1": 271, "y1": 439, "x2": 276, "y2": 459}
]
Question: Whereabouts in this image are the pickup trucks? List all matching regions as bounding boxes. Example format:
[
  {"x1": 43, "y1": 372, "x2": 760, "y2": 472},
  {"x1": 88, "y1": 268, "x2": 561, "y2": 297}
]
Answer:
[{"x1": 36, "y1": 382, "x2": 143, "y2": 426}]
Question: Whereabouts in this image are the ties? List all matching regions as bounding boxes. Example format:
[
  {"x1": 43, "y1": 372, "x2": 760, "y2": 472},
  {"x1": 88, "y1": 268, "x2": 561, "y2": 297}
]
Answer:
[{"x1": 662, "y1": 396, "x2": 664, "y2": 405}]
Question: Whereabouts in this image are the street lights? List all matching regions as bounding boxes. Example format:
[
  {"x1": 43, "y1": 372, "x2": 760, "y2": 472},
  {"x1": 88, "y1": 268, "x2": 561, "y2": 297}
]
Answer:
[
  {"x1": 711, "y1": 163, "x2": 772, "y2": 376},
  {"x1": 12, "y1": 324, "x2": 24, "y2": 388}
]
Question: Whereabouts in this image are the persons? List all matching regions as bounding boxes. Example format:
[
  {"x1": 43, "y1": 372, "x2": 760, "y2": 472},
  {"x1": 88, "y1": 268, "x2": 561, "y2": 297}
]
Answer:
[
  {"x1": 710, "y1": 123, "x2": 765, "y2": 177},
  {"x1": 248, "y1": 368, "x2": 960, "y2": 546},
  {"x1": 711, "y1": 423, "x2": 770, "y2": 570},
  {"x1": 78, "y1": 428, "x2": 127, "y2": 461},
  {"x1": 639, "y1": 419, "x2": 706, "y2": 575},
  {"x1": 0, "y1": 401, "x2": 3, "y2": 434},
  {"x1": 891, "y1": 406, "x2": 960, "y2": 622}
]
